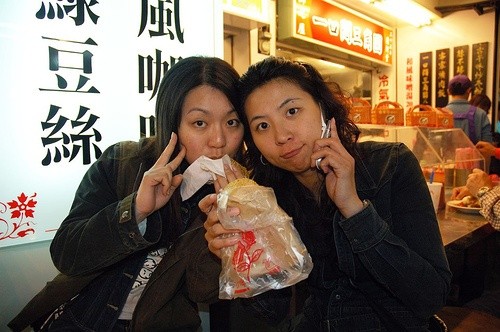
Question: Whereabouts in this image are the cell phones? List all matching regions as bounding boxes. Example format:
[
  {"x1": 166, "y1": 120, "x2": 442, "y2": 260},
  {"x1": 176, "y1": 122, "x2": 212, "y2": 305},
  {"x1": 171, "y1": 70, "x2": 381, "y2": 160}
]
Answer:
[{"x1": 316, "y1": 102, "x2": 331, "y2": 172}]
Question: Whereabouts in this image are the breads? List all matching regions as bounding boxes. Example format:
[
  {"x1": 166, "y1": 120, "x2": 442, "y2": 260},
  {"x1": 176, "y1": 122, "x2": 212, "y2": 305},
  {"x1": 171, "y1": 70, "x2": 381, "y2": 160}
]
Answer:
[
  {"x1": 231, "y1": 158, "x2": 250, "y2": 179},
  {"x1": 219, "y1": 177, "x2": 305, "y2": 281}
]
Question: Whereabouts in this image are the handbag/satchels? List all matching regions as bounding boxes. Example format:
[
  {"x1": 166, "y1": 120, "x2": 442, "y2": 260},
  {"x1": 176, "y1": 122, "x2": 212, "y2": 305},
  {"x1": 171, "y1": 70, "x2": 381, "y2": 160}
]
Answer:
[{"x1": 7, "y1": 273, "x2": 102, "y2": 332}]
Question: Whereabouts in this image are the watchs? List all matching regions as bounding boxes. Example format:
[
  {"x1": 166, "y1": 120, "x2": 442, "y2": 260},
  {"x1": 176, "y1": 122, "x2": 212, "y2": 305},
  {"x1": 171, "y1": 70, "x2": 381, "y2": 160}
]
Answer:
[{"x1": 478, "y1": 186, "x2": 491, "y2": 197}]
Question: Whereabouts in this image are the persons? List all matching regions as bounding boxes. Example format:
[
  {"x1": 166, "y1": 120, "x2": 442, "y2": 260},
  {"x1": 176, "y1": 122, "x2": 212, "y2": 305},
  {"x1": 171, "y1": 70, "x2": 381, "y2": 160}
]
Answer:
[
  {"x1": 428, "y1": 76, "x2": 500, "y2": 231},
  {"x1": 199, "y1": 56, "x2": 451, "y2": 332},
  {"x1": 6, "y1": 56, "x2": 249, "y2": 332}
]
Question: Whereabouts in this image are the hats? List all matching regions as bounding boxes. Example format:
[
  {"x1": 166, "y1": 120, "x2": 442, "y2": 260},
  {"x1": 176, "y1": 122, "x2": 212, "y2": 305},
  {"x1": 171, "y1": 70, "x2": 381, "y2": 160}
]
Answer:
[{"x1": 448, "y1": 75, "x2": 472, "y2": 94}]
